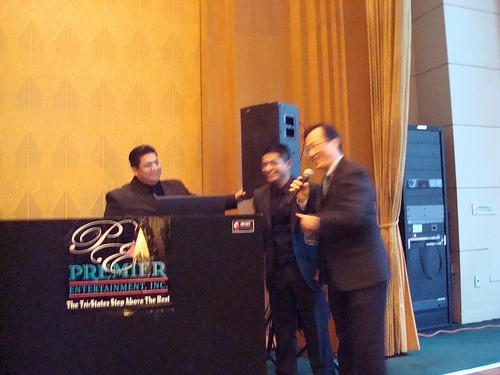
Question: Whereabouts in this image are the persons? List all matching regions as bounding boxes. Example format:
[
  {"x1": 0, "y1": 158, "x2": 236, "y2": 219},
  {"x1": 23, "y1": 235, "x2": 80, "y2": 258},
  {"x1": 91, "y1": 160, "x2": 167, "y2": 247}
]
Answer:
[
  {"x1": 104, "y1": 146, "x2": 246, "y2": 218},
  {"x1": 253, "y1": 143, "x2": 336, "y2": 375},
  {"x1": 288, "y1": 125, "x2": 391, "y2": 375}
]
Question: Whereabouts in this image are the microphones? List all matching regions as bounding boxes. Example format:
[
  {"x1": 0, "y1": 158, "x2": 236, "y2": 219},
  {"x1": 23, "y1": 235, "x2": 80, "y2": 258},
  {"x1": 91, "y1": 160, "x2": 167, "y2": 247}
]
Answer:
[{"x1": 286, "y1": 168, "x2": 313, "y2": 205}]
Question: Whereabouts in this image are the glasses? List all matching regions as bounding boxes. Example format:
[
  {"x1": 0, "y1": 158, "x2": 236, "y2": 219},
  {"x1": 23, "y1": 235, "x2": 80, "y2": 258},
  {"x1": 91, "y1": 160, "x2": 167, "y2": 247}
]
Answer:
[{"x1": 303, "y1": 138, "x2": 334, "y2": 156}]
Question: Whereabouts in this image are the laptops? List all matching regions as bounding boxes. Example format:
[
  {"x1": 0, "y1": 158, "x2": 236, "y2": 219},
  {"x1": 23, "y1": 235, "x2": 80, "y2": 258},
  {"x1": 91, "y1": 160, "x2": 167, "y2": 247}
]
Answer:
[{"x1": 157, "y1": 195, "x2": 225, "y2": 215}]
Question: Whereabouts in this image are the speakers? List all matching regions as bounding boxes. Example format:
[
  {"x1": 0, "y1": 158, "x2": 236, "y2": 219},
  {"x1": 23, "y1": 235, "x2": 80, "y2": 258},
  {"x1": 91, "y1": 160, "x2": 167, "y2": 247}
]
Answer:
[{"x1": 241, "y1": 102, "x2": 299, "y2": 199}]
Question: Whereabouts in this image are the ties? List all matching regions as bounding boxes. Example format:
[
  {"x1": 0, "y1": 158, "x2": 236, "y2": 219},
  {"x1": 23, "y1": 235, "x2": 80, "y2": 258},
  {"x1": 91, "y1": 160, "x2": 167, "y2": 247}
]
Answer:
[{"x1": 321, "y1": 175, "x2": 330, "y2": 197}]
